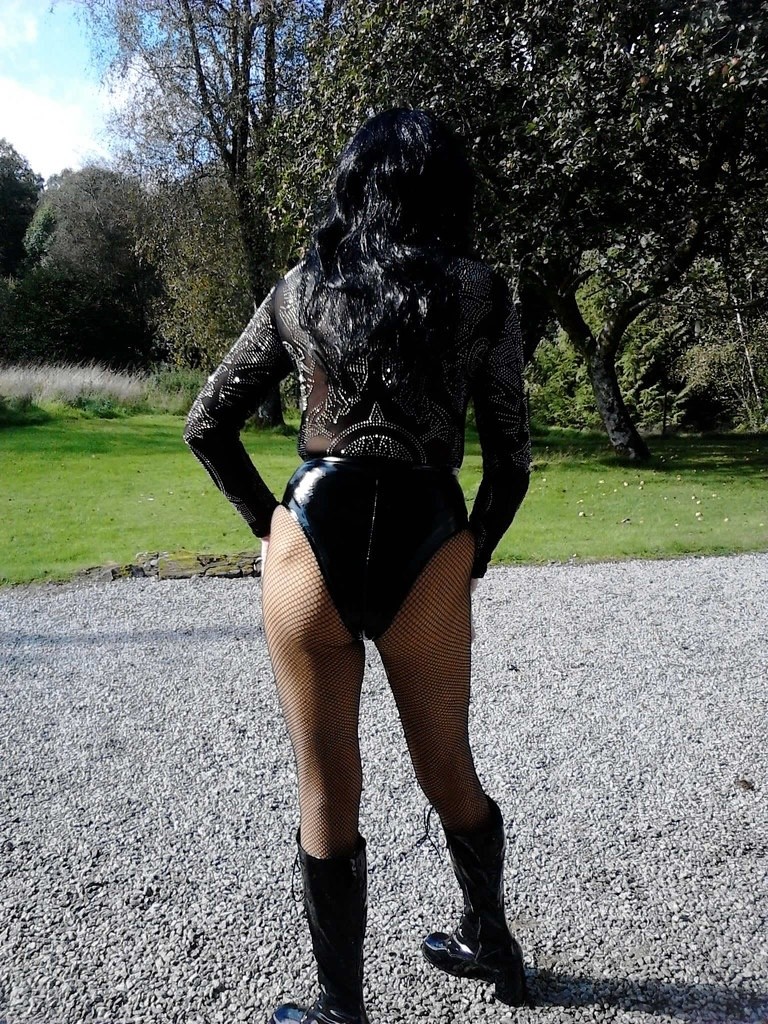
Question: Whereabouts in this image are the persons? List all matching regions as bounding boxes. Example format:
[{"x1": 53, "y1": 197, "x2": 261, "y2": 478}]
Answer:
[{"x1": 185, "y1": 108, "x2": 535, "y2": 1023}]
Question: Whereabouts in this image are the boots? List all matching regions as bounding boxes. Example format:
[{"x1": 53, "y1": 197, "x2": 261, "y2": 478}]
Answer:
[
  {"x1": 268, "y1": 820, "x2": 369, "y2": 1024},
  {"x1": 422, "y1": 793, "x2": 529, "y2": 1008}
]
